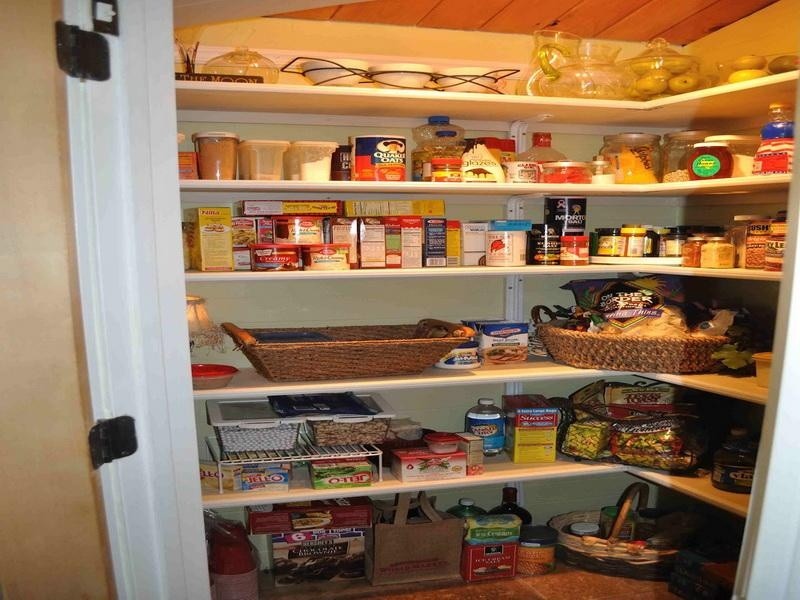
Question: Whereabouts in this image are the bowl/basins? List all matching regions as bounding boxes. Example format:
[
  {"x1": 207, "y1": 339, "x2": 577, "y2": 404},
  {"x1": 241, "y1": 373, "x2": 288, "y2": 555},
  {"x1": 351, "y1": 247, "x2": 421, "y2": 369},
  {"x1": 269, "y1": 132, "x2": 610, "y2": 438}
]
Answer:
[
  {"x1": 301, "y1": 59, "x2": 368, "y2": 87},
  {"x1": 432, "y1": 68, "x2": 498, "y2": 93},
  {"x1": 365, "y1": 63, "x2": 434, "y2": 91}
]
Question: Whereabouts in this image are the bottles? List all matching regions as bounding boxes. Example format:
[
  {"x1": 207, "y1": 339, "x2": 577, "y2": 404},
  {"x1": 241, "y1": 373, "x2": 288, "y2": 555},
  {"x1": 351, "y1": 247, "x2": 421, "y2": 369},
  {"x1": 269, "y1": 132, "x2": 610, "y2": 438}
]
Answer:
[
  {"x1": 517, "y1": 28, "x2": 712, "y2": 109},
  {"x1": 201, "y1": 44, "x2": 281, "y2": 85},
  {"x1": 445, "y1": 487, "x2": 532, "y2": 526},
  {"x1": 411, "y1": 116, "x2": 466, "y2": 182},
  {"x1": 464, "y1": 399, "x2": 505, "y2": 456}
]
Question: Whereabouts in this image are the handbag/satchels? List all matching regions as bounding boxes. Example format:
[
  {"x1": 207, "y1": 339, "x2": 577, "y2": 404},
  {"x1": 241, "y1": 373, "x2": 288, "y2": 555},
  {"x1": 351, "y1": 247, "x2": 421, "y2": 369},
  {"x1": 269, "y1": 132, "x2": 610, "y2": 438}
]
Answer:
[{"x1": 360, "y1": 489, "x2": 464, "y2": 586}]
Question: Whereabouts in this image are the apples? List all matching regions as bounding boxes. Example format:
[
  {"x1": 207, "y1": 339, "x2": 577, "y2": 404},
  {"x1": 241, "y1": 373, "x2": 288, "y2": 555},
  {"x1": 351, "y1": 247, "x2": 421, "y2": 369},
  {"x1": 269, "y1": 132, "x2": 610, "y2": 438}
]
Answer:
[
  {"x1": 728, "y1": 54, "x2": 799, "y2": 85},
  {"x1": 638, "y1": 53, "x2": 698, "y2": 100}
]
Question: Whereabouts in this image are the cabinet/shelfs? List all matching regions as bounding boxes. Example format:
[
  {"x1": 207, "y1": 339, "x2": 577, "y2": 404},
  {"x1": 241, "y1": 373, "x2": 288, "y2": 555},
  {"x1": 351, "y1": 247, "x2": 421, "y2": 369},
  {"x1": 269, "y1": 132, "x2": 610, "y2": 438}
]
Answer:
[{"x1": 171, "y1": 74, "x2": 800, "y2": 523}]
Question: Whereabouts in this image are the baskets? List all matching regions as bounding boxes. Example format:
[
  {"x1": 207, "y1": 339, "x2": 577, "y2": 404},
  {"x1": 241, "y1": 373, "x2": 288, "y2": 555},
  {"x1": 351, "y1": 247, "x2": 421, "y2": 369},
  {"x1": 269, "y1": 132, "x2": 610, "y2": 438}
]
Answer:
[
  {"x1": 545, "y1": 479, "x2": 703, "y2": 581},
  {"x1": 529, "y1": 303, "x2": 732, "y2": 375},
  {"x1": 218, "y1": 319, "x2": 475, "y2": 383}
]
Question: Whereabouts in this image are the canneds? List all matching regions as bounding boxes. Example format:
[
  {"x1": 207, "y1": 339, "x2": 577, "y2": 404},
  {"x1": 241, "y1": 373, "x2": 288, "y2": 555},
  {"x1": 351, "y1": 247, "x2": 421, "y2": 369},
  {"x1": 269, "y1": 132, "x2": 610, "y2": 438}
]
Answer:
[
  {"x1": 430, "y1": 158, "x2": 462, "y2": 182},
  {"x1": 570, "y1": 505, "x2": 659, "y2": 540},
  {"x1": 595, "y1": 213, "x2": 786, "y2": 271},
  {"x1": 249, "y1": 243, "x2": 304, "y2": 271},
  {"x1": 306, "y1": 243, "x2": 351, "y2": 269},
  {"x1": 350, "y1": 134, "x2": 407, "y2": 181},
  {"x1": 560, "y1": 234, "x2": 591, "y2": 266},
  {"x1": 485, "y1": 230, "x2": 527, "y2": 267}
]
